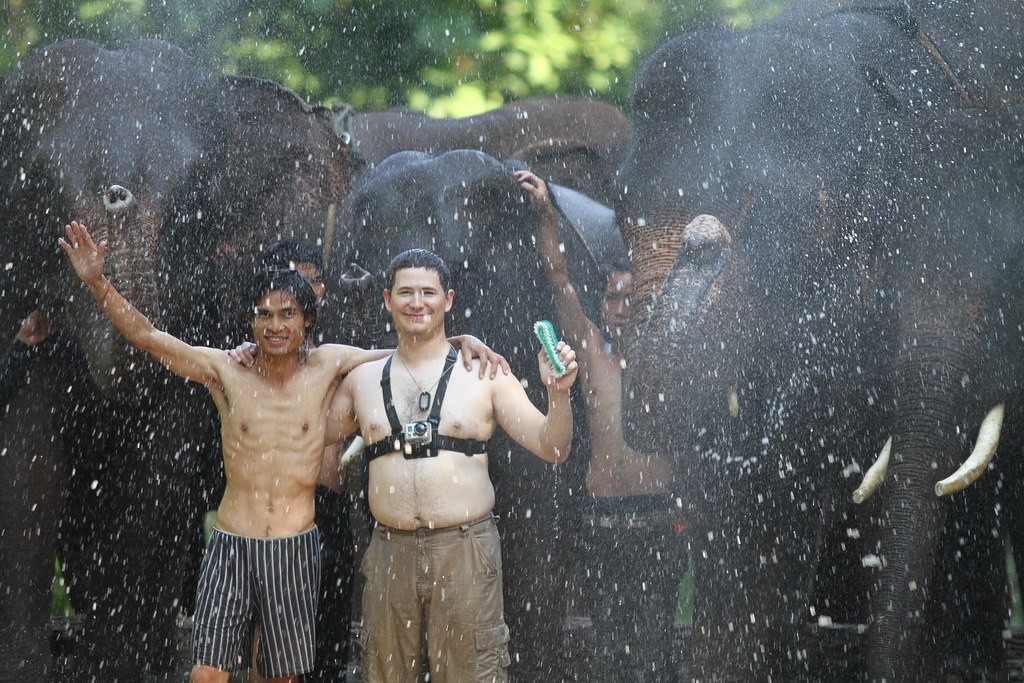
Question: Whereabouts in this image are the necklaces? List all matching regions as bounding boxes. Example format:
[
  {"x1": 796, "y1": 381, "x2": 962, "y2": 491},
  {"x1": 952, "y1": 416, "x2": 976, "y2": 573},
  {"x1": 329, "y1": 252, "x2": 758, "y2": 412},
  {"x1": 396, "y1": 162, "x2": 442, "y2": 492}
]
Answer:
[{"x1": 396, "y1": 345, "x2": 459, "y2": 412}]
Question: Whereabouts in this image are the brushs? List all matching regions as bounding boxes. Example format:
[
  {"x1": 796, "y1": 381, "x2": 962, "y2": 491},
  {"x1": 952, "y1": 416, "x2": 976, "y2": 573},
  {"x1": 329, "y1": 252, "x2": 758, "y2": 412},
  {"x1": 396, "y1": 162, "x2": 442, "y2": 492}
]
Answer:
[{"x1": 530, "y1": 319, "x2": 568, "y2": 380}]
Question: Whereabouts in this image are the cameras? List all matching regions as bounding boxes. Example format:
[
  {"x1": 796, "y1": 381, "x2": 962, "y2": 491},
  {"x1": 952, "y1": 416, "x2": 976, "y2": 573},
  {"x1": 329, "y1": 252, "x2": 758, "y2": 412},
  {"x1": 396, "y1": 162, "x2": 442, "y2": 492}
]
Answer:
[{"x1": 405, "y1": 421, "x2": 432, "y2": 445}]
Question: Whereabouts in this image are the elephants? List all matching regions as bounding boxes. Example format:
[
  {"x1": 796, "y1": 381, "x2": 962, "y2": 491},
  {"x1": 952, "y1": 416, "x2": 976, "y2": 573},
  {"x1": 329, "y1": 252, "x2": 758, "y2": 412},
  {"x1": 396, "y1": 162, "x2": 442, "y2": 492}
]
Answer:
[{"x1": 1, "y1": 0, "x2": 1023, "y2": 682}]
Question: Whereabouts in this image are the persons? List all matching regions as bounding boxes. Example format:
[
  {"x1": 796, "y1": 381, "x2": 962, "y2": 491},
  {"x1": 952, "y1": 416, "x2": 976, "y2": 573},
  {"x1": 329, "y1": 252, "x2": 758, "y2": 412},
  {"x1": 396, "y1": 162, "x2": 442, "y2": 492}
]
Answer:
[
  {"x1": 515, "y1": 170, "x2": 688, "y2": 683},
  {"x1": 252, "y1": 238, "x2": 325, "y2": 683},
  {"x1": 58, "y1": 223, "x2": 510, "y2": 682},
  {"x1": 229, "y1": 248, "x2": 577, "y2": 683}
]
